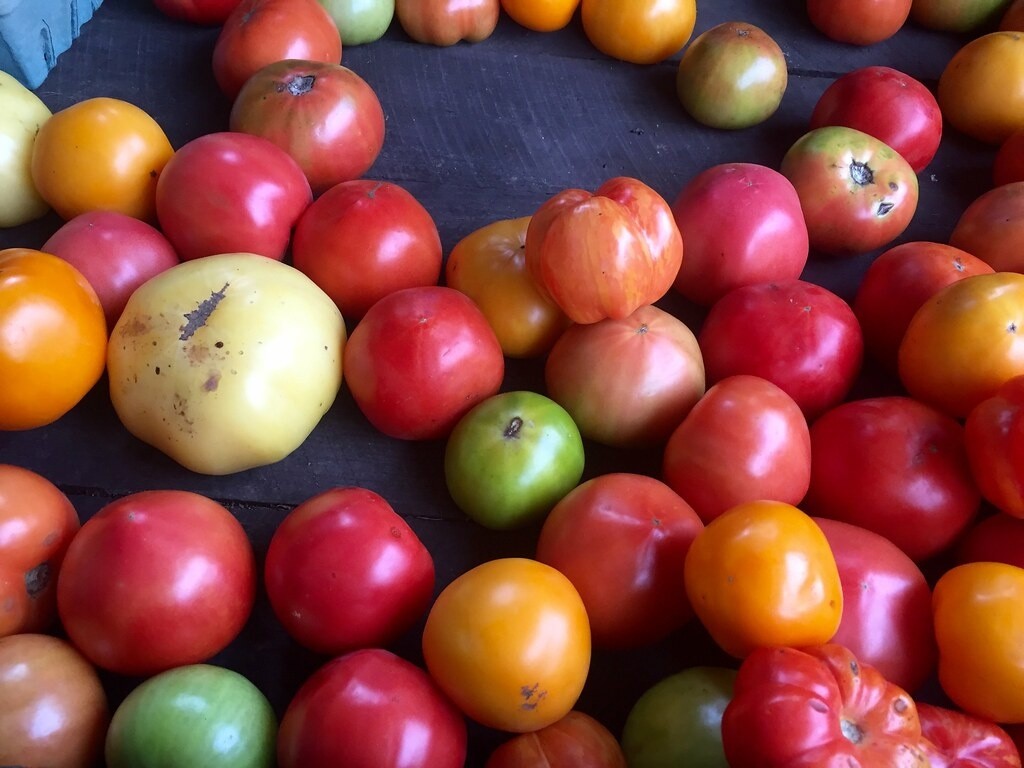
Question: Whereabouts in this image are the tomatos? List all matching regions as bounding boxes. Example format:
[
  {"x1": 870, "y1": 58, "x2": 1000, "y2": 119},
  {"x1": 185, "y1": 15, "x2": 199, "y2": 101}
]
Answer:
[{"x1": 0, "y1": 0, "x2": 1024, "y2": 768}]
